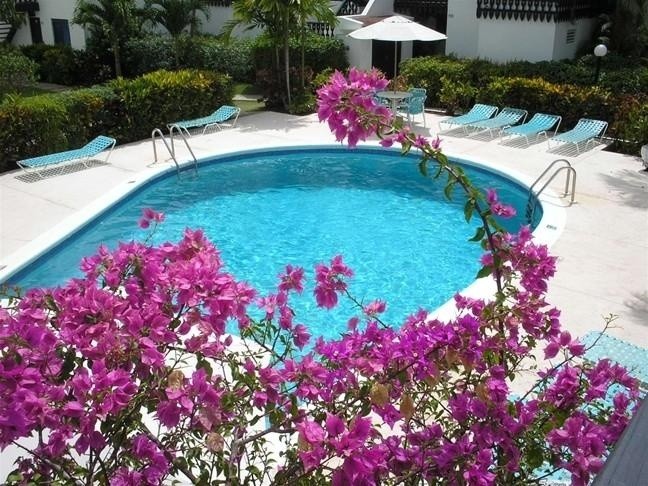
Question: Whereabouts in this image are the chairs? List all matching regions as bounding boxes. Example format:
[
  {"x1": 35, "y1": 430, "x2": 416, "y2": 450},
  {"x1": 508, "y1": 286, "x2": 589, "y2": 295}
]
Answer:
[
  {"x1": 439, "y1": 103, "x2": 499, "y2": 136},
  {"x1": 166, "y1": 105, "x2": 242, "y2": 138},
  {"x1": 465, "y1": 108, "x2": 528, "y2": 138},
  {"x1": 499, "y1": 113, "x2": 563, "y2": 146},
  {"x1": 17, "y1": 135, "x2": 116, "y2": 179},
  {"x1": 372, "y1": 89, "x2": 427, "y2": 129},
  {"x1": 548, "y1": 119, "x2": 609, "y2": 155},
  {"x1": 487, "y1": 331, "x2": 648, "y2": 486}
]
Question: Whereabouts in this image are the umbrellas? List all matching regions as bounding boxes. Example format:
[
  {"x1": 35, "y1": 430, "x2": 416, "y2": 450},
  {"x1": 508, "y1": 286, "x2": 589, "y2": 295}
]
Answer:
[{"x1": 345, "y1": 14, "x2": 448, "y2": 95}]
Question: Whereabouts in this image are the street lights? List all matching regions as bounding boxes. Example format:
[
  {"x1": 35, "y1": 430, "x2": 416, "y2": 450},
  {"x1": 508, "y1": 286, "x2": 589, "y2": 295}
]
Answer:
[{"x1": 594, "y1": 44, "x2": 608, "y2": 82}]
